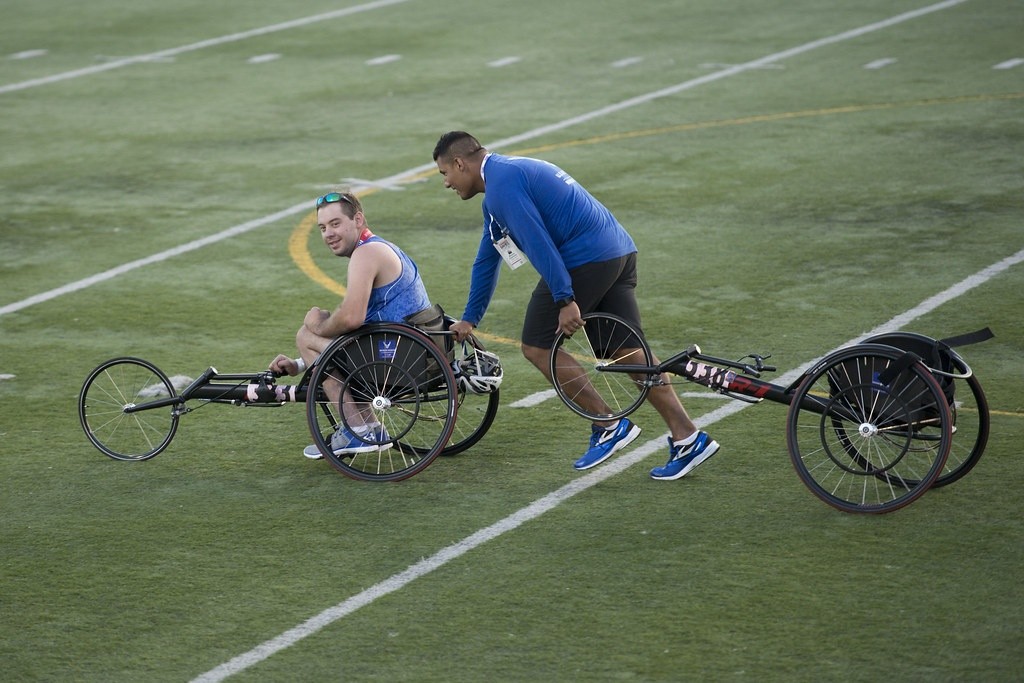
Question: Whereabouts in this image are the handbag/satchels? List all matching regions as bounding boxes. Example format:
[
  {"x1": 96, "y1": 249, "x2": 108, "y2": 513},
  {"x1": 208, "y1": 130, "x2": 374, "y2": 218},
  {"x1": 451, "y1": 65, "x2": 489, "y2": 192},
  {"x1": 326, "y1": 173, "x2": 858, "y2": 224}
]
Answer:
[{"x1": 829, "y1": 331, "x2": 955, "y2": 430}]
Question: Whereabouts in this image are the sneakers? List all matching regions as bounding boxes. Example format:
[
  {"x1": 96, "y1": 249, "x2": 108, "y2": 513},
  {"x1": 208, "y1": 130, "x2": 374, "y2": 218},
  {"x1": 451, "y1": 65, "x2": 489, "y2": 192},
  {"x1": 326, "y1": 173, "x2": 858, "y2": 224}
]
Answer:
[
  {"x1": 574, "y1": 418, "x2": 642, "y2": 470},
  {"x1": 650, "y1": 430, "x2": 720, "y2": 481},
  {"x1": 374, "y1": 423, "x2": 393, "y2": 451}
]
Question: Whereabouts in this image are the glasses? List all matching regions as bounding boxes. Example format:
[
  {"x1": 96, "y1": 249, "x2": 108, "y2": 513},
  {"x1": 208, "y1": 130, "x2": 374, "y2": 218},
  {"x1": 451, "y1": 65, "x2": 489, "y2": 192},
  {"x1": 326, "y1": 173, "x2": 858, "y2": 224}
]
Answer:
[{"x1": 317, "y1": 192, "x2": 357, "y2": 210}]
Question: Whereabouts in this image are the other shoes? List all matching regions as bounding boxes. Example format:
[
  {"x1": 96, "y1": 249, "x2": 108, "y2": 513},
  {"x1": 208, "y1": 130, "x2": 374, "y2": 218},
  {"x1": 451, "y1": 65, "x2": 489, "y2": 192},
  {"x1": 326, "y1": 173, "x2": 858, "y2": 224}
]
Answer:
[{"x1": 303, "y1": 422, "x2": 378, "y2": 459}]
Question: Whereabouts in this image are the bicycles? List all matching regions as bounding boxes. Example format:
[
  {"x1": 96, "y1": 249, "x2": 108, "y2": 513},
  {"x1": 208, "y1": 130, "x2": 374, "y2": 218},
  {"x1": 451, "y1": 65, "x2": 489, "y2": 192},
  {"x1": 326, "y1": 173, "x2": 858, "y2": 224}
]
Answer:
[
  {"x1": 78, "y1": 301, "x2": 500, "y2": 483},
  {"x1": 549, "y1": 312, "x2": 994, "y2": 515}
]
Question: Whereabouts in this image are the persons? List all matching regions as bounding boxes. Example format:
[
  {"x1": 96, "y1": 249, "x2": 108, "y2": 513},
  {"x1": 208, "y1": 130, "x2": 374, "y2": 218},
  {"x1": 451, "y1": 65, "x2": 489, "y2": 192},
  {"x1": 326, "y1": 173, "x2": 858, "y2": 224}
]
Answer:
[
  {"x1": 431, "y1": 131, "x2": 723, "y2": 482},
  {"x1": 267, "y1": 192, "x2": 455, "y2": 459}
]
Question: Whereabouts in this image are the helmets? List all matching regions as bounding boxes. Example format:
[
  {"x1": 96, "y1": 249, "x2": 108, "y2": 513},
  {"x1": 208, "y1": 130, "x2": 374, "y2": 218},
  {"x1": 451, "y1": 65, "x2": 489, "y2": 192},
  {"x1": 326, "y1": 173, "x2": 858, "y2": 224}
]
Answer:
[{"x1": 452, "y1": 350, "x2": 503, "y2": 396}]
